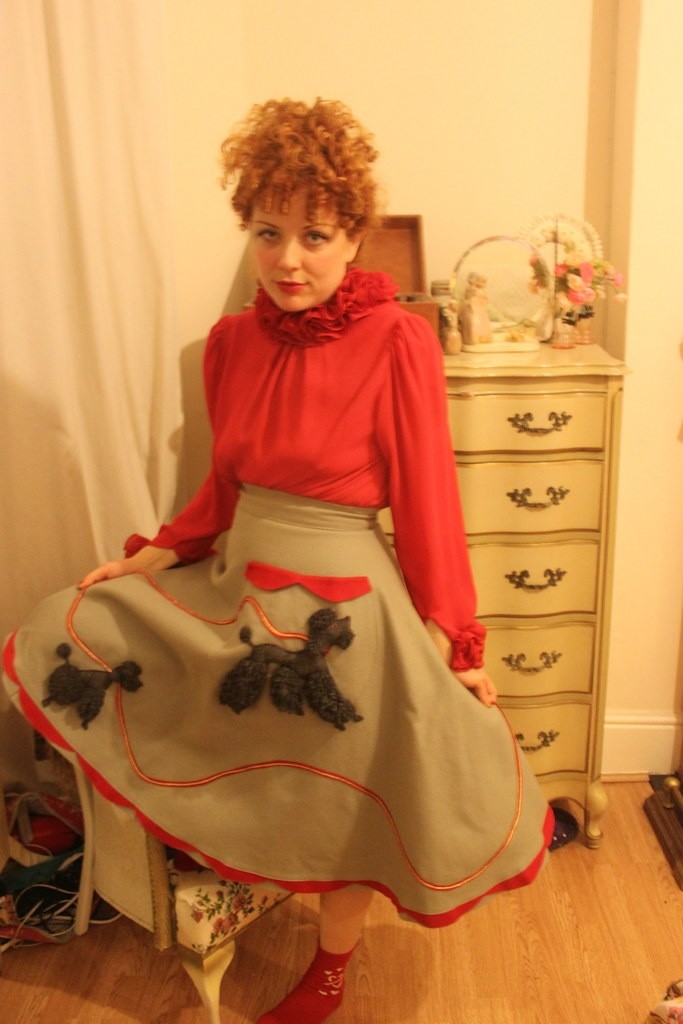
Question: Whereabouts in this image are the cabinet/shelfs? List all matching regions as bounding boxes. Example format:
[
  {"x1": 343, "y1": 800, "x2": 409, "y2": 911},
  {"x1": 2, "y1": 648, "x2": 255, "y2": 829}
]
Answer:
[{"x1": 378, "y1": 339, "x2": 632, "y2": 851}]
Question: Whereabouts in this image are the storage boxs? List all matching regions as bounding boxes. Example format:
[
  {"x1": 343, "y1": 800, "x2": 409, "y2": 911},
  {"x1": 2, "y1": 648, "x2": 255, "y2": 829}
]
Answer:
[{"x1": 243, "y1": 214, "x2": 440, "y2": 342}]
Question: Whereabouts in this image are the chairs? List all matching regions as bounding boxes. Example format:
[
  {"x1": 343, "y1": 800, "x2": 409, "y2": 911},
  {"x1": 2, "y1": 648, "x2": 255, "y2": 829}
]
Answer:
[{"x1": 71, "y1": 763, "x2": 297, "y2": 1024}]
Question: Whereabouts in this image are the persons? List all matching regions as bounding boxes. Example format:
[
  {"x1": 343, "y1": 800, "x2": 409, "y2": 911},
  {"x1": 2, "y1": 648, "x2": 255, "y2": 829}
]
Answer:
[{"x1": 2, "y1": 97, "x2": 555, "y2": 1024}]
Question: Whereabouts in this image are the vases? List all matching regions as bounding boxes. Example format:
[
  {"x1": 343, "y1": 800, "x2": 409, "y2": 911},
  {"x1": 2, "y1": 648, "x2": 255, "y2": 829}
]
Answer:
[{"x1": 551, "y1": 316, "x2": 597, "y2": 348}]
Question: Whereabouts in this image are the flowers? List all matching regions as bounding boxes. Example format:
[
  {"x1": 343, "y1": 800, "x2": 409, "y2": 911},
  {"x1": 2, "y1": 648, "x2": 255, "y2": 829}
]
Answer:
[
  {"x1": 528, "y1": 256, "x2": 548, "y2": 292},
  {"x1": 554, "y1": 252, "x2": 623, "y2": 327}
]
